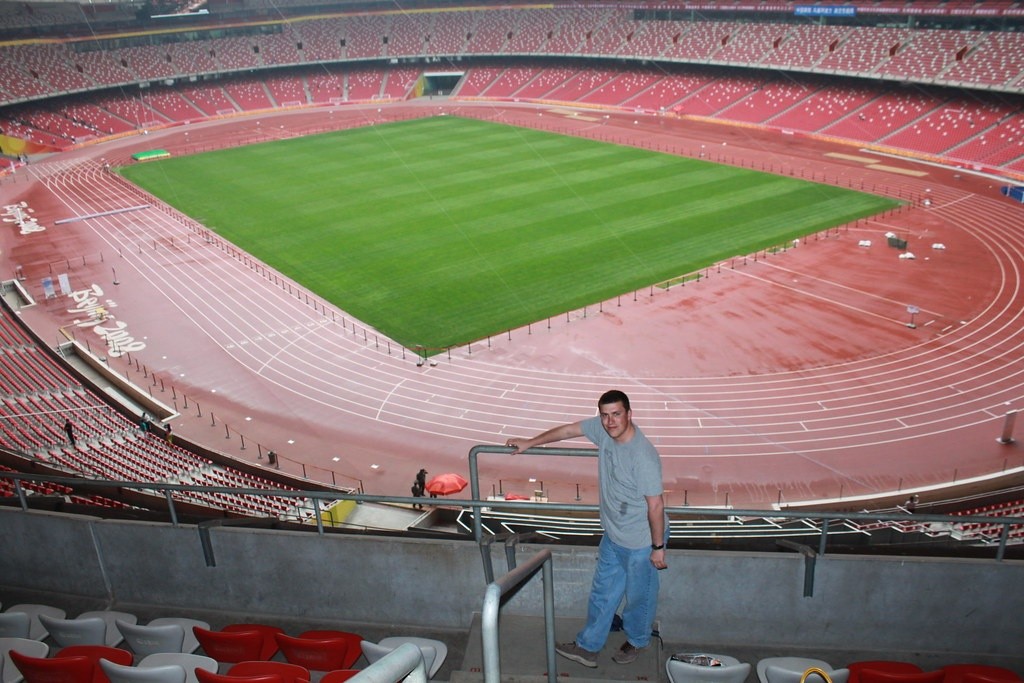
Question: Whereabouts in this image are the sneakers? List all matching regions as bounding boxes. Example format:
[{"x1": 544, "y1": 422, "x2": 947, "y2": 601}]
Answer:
[
  {"x1": 611, "y1": 640, "x2": 654, "y2": 664},
  {"x1": 554, "y1": 640, "x2": 599, "y2": 669}
]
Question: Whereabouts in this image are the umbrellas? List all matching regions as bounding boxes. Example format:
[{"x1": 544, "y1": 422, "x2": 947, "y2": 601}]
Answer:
[{"x1": 424, "y1": 472, "x2": 468, "y2": 499}]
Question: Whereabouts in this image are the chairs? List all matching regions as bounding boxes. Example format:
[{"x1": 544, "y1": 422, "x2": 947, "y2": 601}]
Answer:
[
  {"x1": 0, "y1": 600, "x2": 449, "y2": 683},
  {"x1": 666, "y1": 651, "x2": 1024, "y2": 683},
  {"x1": 860, "y1": 499, "x2": 1024, "y2": 538},
  {"x1": 0, "y1": 305, "x2": 333, "y2": 524}
]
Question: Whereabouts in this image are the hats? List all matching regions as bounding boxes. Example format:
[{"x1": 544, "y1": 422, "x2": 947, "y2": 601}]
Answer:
[{"x1": 420, "y1": 469, "x2": 428, "y2": 474}]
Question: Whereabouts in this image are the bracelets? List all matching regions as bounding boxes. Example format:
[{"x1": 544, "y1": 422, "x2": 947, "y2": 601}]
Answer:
[{"x1": 651, "y1": 543, "x2": 664, "y2": 550}]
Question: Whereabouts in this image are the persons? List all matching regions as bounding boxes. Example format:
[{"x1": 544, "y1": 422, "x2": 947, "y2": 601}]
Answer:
[
  {"x1": 139, "y1": 412, "x2": 149, "y2": 431},
  {"x1": 163, "y1": 423, "x2": 173, "y2": 443},
  {"x1": 504, "y1": 389, "x2": 670, "y2": 668},
  {"x1": 411, "y1": 468, "x2": 437, "y2": 510},
  {"x1": 906, "y1": 496, "x2": 915, "y2": 513},
  {"x1": 64, "y1": 418, "x2": 76, "y2": 449}
]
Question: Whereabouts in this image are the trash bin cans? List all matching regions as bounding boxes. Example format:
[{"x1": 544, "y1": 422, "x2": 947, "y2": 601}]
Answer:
[
  {"x1": 888, "y1": 238, "x2": 906, "y2": 250},
  {"x1": 268, "y1": 452, "x2": 276, "y2": 463}
]
